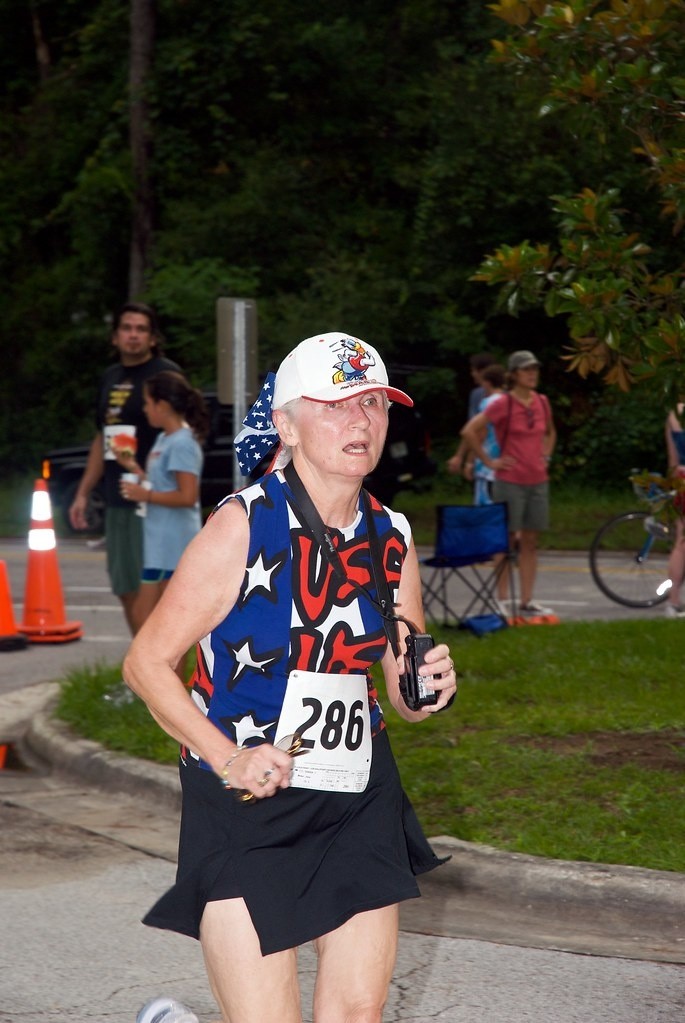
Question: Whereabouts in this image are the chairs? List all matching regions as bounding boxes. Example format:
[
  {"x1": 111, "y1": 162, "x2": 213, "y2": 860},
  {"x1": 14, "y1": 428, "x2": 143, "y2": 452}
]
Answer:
[{"x1": 418, "y1": 501, "x2": 518, "y2": 632}]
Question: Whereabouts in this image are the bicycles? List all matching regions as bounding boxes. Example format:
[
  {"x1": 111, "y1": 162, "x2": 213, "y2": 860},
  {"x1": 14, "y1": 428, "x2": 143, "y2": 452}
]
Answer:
[{"x1": 588, "y1": 470, "x2": 685, "y2": 607}]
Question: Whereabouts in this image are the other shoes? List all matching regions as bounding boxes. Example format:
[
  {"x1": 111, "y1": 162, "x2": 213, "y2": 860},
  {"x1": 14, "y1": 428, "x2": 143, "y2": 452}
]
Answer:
[
  {"x1": 499, "y1": 598, "x2": 516, "y2": 618},
  {"x1": 519, "y1": 598, "x2": 554, "y2": 617},
  {"x1": 136, "y1": 997, "x2": 199, "y2": 1023},
  {"x1": 666, "y1": 602, "x2": 685, "y2": 618}
]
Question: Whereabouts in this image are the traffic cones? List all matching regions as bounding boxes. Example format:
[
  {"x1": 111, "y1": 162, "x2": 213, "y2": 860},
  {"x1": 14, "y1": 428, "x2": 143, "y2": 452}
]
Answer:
[
  {"x1": 17, "y1": 629, "x2": 85, "y2": 643},
  {"x1": 0, "y1": 560, "x2": 25, "y2": 652},
  {"x1": 15, "y1": 478, "x2": 86, "y2": 633}
]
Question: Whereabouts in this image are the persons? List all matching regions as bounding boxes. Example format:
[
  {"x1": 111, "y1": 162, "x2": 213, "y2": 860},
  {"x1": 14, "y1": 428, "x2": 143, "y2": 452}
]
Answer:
[
  {"x1": 666, "y1": 402, "x2": 685, "y2": 615},
  {"x1": 449, "y1": 352, "x2": 521, "y2": 567},
  {"x1": 461, "y1": 351, "x2": 557, "y2": 616},
  {"x1": 109, "y1": 372, "x2": 206, "y2": 679},
  {"x1": 69, "y1": 305, "x2": 183, "y2": 629},
  {"x1": 121, "y1": 333, "x2": 456, "y2": 1022}
]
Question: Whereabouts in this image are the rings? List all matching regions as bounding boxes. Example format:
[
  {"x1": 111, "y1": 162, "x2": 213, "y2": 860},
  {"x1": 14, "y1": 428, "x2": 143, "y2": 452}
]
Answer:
[
  {"x1": 258, "y1": 779, "x2": 269, "y2": 788},
  {"x1": 446, "y1": 658, "x2": 454, "y2": 672},
  {"x1": 263, "y1": 766, "x2": 275, "y2": 777}
]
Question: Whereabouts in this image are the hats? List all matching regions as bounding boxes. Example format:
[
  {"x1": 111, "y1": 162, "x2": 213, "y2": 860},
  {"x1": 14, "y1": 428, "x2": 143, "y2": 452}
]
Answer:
[
  {"x1": 270, "y1": 331, "x2": 414, "y2": 411},
  {"x1": 508, "y1": 350, "x2": 540, "y2": 372}
]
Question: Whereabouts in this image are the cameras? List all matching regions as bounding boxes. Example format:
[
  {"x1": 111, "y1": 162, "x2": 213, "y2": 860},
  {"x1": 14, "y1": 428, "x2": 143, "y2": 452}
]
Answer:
[{"x1": 399, "y1": 633, "x2": 442, "y2": 711}]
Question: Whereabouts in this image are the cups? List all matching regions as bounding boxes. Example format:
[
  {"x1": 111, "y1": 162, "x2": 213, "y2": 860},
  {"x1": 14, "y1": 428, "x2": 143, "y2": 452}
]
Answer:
[{"x1": 122, "y1": 472, "x2": 139, "y2": 487}]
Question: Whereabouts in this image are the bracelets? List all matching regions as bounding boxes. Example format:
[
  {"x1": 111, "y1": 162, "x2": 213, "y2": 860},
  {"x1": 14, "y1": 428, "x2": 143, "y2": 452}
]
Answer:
[
  {"x1": 148, "y1": 489, "x2": 153, "y2": 503},
  {"x1": 544, "y1": 456, "x2": 552, "y2": 461},
  {"x1": 221, "y1": 745, "x2": 248, "y2": 790}
]
